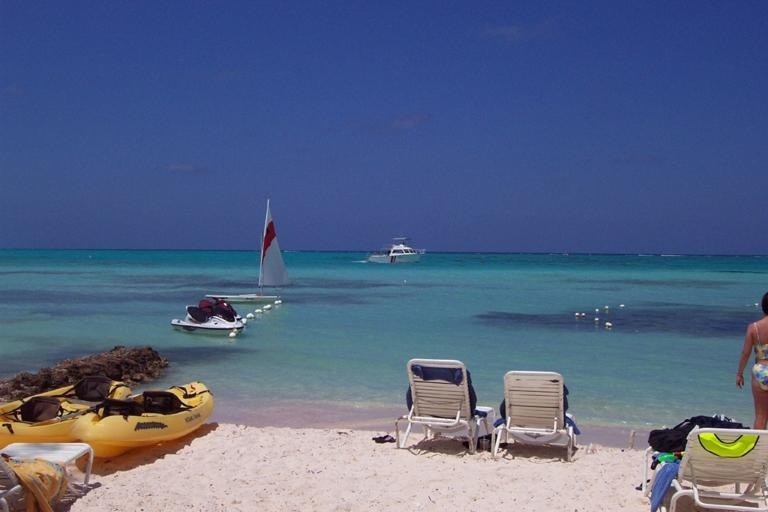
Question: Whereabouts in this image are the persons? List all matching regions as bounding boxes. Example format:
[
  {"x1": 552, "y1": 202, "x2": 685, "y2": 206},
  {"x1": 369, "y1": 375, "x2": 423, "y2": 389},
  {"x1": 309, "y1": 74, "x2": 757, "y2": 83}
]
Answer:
[{"x1": 735, "y1": 292, "x2": 768, "y2": 432}]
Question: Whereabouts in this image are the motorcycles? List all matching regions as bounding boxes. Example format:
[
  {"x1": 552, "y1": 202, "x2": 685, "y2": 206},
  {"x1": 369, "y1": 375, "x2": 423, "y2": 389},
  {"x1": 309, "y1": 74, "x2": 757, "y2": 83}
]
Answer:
[{"x1": 170, "y1": 296, "x2": 245, "y2": 332}]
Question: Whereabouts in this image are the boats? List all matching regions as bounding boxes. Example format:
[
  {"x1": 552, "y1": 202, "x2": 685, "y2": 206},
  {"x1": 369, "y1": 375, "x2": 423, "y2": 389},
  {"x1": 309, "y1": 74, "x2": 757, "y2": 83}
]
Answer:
[
  {"x1": 74, "y1": 380, "x2": 216, "y2": 462},
  {"x1": 368, "y1": 236, "x2": 424, "y2": 264},
  {"x1": 0, "y1": 373, "x2": 135, "y2": 468}
]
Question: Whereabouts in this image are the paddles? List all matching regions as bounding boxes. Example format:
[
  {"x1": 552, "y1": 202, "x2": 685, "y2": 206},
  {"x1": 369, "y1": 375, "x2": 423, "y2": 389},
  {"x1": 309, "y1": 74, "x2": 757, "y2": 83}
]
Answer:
[{"x1": 32, "y1": 394, "x2": 144, "y2": 426}]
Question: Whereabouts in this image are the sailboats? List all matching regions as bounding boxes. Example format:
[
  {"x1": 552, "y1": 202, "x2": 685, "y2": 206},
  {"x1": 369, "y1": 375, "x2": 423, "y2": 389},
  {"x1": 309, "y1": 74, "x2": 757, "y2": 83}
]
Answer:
[{"x1": 202, "y1": 197, "x2": 293, "y2": 303}]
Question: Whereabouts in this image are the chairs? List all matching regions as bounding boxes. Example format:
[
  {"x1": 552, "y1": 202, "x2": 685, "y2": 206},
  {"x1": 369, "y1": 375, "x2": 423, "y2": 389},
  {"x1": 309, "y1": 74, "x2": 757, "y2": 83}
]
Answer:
[
  {"x1": 643, "y1": 428, "x2": 767, "y2": 512},
  {"x1": 393, "y1": 358, "x2": 496, "y2": 454},
  {"x1": 490, "y1": 371, "x2": 578, "y2": 461},
  {"x1": 0, "y1": 442, "x2": 95, "y2": 512}
]
